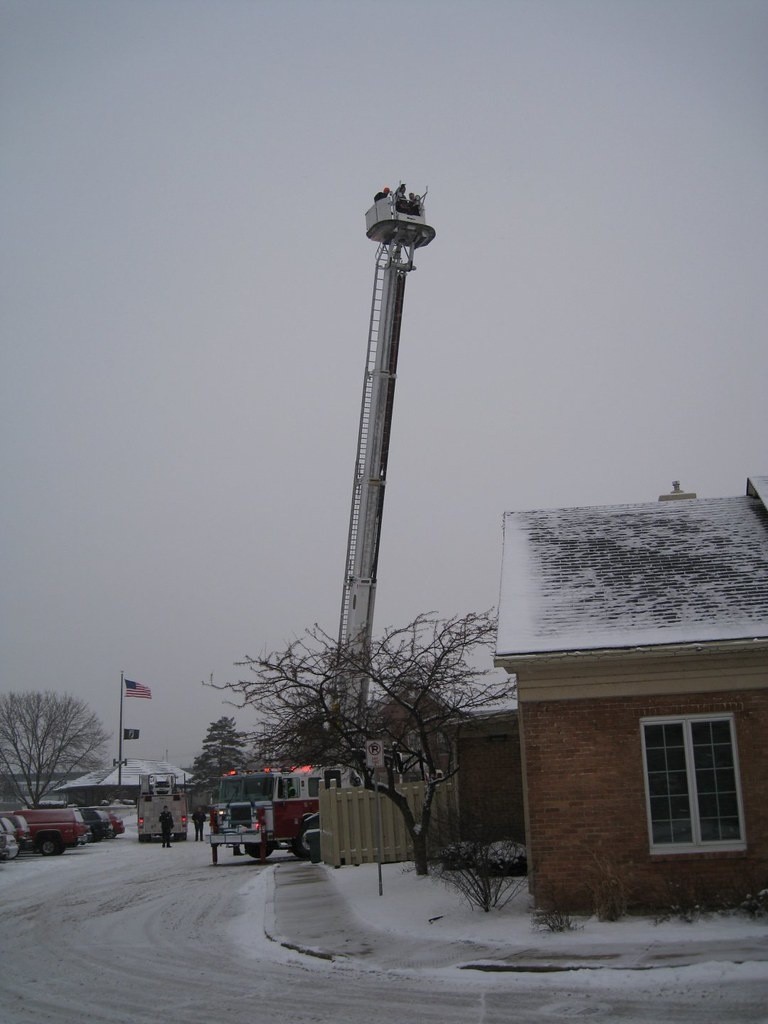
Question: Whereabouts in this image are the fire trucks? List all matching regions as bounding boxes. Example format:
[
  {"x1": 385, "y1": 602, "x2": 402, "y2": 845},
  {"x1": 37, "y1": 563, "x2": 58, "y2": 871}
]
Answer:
[{"x1": 205, "y1": 179, "x2": 436, "y2": 866}]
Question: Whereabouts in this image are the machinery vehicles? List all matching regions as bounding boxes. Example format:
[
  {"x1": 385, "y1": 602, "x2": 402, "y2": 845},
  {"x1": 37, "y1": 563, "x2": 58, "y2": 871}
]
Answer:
[{"x1": 137, "y1": 772, "x2": 188, "y2": 841}]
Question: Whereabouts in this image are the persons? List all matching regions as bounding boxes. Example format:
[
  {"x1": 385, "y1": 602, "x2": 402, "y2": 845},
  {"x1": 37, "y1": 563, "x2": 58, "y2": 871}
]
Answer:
[
  {"x1": 192, "y1": 806, "x2": 206, "y2": 841},
  {"x1": 159, "y1": 805, "x2": 174, "y2": 848}
]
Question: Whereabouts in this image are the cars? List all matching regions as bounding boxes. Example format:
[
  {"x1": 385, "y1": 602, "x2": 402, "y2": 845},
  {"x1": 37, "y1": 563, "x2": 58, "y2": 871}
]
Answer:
[
  {"x1": 98, "y1": 810, "x2": 125, "y2": 838},
  {"x1": 0, "y1": 803, "x2": 89, "y2": 855},
  {"x1": 0, "y1": 832, "x2": 20, "y2": 860},
  {"x1": 77, "y1": 807, "x2": 112, "y2": 843}
]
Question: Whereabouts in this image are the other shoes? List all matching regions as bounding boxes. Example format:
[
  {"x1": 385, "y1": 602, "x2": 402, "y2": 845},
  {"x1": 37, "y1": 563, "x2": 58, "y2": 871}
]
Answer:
[
  {"x1": 162, "y1": 845, "x2": 165, "y2": 847},
  {"x1": 167, "y1": 845, "x2": 172, "y2": 847}
]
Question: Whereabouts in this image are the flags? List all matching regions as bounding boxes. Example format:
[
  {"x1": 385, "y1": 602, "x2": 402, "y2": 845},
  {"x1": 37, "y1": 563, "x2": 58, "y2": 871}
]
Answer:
[
  {"x1": 124, "y1": 729, "x2": 139, "y2": 739},
  {"x1": 125, "y1": 680, "x2": 150, "y2": 698}
]
